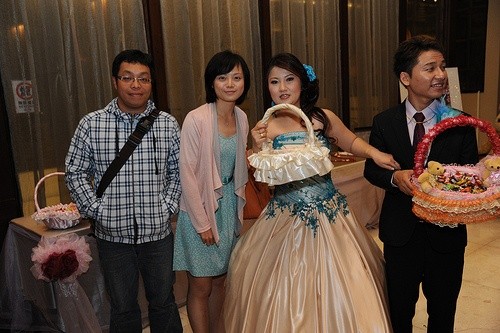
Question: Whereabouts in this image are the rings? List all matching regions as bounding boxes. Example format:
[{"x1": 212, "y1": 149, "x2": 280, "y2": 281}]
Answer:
[{"x1": 260, "y1": 132, "x2": 269, "y2": 153}]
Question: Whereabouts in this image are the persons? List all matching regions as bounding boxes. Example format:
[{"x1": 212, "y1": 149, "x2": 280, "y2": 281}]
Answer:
[
  {"x1": 364, "y1": 37, "x2": 478, "y2": 333},
  {"x1": 250, "y1": 51, "x2": 401, "y2": 333},
  {"x1": 178, "y1": 51, "x2": 251, "y2": 333},
  {"x1": 65, "y1": 50, "x2": 184, "y2": 333}
]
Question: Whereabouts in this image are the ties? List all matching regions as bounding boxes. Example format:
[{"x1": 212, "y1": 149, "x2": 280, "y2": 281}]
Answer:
[{"x1": 413, "y1": 112, "x2": 424, "y2": 165}]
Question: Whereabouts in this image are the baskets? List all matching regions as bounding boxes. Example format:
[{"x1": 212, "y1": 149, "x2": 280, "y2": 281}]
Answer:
[
  {"x1": 250, "y1": 104, "x2": 334, "y2": 186},
  {"x1": 412, "y1": 116, "x2": 500, "y2": 227},
  {"x1": 33, "y1": 172, "x2": 83, "y2": 230}
]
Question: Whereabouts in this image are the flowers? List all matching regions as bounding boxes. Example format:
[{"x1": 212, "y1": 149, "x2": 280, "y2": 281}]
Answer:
[
  {"x1": 303, "y1": 64, "x2": 316, "y2": 82},
  {"x1": 40, "y1": 249, "x2": 79, "y2": 281},
  {"x1": 428, "y1": 95, "x2": 460, "y2": 123}
]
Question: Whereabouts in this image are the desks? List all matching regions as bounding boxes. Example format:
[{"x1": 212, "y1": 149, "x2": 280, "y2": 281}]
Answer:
[{"x1": 9, "y1": 152, "x2": 384, "y2": 333}]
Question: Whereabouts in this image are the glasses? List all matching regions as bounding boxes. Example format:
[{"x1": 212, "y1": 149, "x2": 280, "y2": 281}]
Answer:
[{"x1": 116, "y1": 75, "x2": 152, "y2": 84}]
